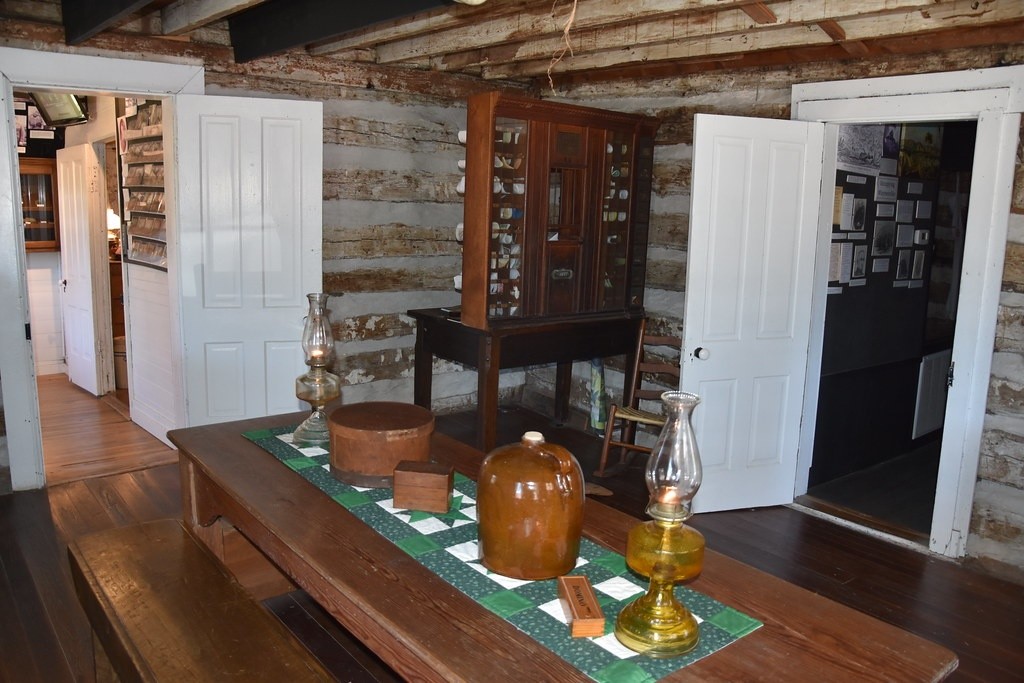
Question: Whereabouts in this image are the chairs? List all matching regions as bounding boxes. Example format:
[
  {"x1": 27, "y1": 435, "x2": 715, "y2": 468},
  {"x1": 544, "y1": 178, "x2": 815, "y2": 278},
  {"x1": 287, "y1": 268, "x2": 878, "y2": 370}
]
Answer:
[{"x1": 594, "y1": 317, "x2": 682, "y2": 479}]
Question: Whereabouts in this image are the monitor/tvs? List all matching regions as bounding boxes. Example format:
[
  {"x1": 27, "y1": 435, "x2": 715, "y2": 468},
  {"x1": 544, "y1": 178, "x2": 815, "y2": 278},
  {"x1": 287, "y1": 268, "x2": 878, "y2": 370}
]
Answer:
[{"x1": 28, "y1": 92, "x2": 88, "y2": 127}]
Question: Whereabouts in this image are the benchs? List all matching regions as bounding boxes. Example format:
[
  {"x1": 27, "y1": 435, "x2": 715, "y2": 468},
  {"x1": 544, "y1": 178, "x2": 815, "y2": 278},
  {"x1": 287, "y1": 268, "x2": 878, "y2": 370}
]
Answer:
[{"x1": 67, "y1": 520, "x2": 336, "y2": 683}]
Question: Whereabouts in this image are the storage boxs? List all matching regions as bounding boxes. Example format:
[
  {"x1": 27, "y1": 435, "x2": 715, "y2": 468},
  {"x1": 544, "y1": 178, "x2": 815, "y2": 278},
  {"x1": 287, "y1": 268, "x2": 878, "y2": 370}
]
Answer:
[{"x1": 393, "y1": 459, "x2": 455, "y2": 513}]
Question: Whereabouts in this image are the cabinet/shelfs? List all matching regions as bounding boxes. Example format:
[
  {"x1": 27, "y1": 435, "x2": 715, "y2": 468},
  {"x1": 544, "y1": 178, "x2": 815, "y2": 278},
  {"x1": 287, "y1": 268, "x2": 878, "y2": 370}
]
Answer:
[
  {"x1": 461, "y1": 87, "x2": 660, "y2": 330},
  {"x1": 122, "y1": 134, "x2": 169, "y2": 273},
  {"x1": 16, "y1": 156, "x2": 61, "y2": 254}
]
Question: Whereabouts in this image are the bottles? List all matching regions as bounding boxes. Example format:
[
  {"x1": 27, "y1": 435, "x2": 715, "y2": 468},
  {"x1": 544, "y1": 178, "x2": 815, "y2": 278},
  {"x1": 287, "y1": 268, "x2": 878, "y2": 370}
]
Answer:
[{"x1": 477, "y1": 432, "x2": 585, "y2": 579}]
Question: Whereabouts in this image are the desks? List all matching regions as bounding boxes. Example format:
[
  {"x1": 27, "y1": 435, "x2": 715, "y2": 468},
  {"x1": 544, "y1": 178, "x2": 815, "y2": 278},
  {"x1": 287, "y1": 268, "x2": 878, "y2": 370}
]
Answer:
[
  {"x1": 166, "y1": 405, "x2": 960, "y2": 683},
  {"x1": 408, "y1": 305, "x2": 646, "y2": 454}
]
